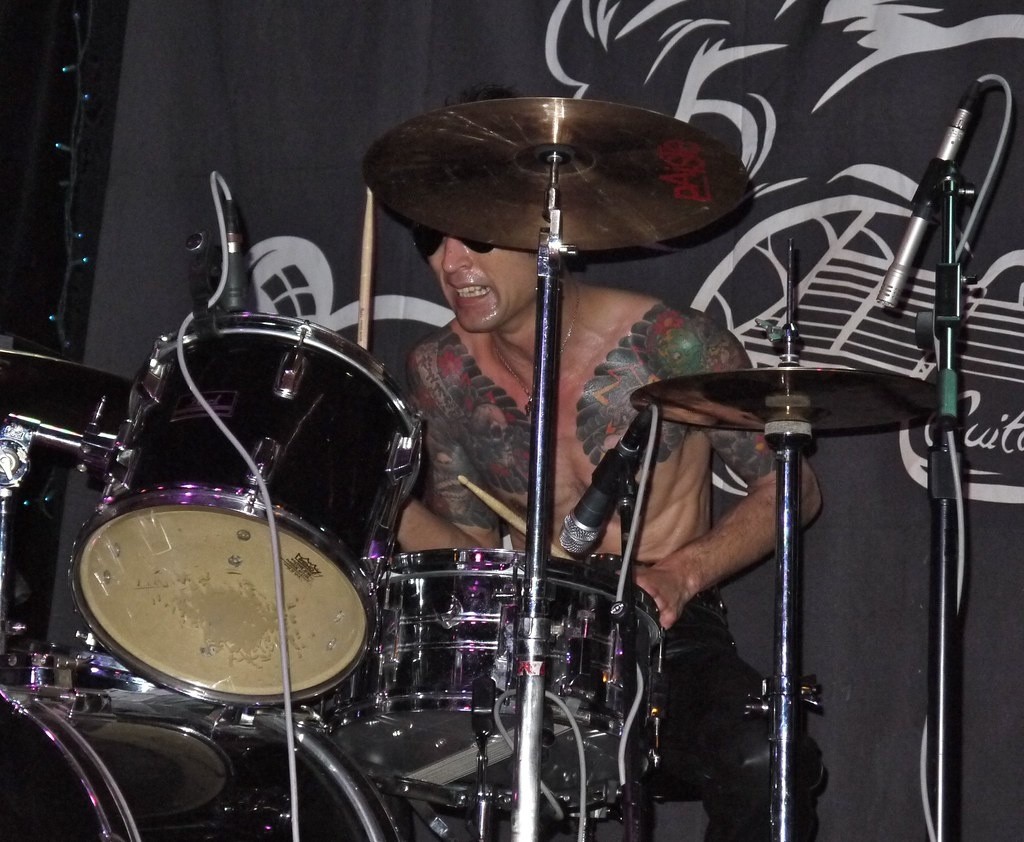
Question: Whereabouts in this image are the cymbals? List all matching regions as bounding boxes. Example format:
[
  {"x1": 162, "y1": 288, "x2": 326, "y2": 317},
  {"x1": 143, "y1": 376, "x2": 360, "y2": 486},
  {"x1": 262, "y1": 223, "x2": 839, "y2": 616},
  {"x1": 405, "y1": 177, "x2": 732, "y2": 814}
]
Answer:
[
  {"x1": 633, "y1": 366, "x2": 939, "y2": 436},
  {"x1": 363, "y1": 95, "x2": 752, "y2": 252}
]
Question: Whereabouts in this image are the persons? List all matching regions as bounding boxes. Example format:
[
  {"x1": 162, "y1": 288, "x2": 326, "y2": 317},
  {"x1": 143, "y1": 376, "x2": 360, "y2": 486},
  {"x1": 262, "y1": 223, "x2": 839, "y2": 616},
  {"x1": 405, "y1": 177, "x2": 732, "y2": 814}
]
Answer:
[{"x1": 397, "y1": 224, "x2": 828, "y2": 842}]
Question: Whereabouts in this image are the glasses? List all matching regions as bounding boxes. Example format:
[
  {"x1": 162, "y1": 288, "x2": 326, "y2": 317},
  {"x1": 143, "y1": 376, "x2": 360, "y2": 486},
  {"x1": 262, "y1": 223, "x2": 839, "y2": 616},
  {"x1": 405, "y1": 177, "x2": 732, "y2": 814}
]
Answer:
[{"x1": 411, "y1": 223, "x2": 494, "y2": 253}]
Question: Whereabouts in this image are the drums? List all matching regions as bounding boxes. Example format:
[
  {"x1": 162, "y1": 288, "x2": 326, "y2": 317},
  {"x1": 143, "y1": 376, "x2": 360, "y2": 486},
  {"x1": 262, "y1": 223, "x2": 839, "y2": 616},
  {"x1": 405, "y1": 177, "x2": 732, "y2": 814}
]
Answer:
[
  {"x1": 70, "y1": 312, "x2": 425, "y2": 697},
  {"x1": 0, "y1": 664, "x2": 398, "y2": 840},
  {"x1": 329, "y1": 549, "x2": 666, "y2": 821}
]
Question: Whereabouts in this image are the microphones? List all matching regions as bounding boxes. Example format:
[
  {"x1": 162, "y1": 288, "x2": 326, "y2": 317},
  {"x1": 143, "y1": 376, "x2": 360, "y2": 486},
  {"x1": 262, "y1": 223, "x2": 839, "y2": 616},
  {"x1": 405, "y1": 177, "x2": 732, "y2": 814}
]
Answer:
[
  {"x1": 221, "y1": 199, "x2": 249, "y2": 313},
  {"x1": 559, "y1": 403, "x2": 654, "y2": 554},
  {"x1": 877, "y1": 79, "x2": 982, "y2": 308}
]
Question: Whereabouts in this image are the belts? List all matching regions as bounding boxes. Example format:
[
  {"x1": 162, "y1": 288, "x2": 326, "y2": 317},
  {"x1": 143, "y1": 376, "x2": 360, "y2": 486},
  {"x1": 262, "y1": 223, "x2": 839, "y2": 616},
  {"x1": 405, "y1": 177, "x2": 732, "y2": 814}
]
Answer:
[{"x1": 589, "y1": 553, "x2": 728, "y2": 625}]
